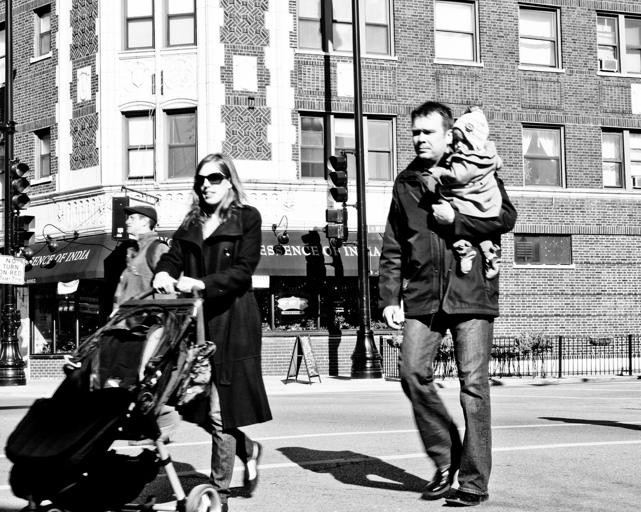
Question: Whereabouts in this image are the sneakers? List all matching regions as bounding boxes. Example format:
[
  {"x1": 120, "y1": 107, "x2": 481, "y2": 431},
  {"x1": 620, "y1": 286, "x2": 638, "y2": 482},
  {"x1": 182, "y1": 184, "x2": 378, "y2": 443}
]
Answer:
[
  {"x1": 485, "y1": 255, "x2": 502, "y2": 278},
  {"x1": 461, "y1": 249, "x2": 477, "y2": 274}
]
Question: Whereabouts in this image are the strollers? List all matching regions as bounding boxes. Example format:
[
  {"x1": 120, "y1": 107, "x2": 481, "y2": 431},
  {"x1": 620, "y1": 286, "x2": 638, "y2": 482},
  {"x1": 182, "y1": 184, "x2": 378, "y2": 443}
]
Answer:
[{"x1": 4, "y1": 288, "x2": 229, "y2": 512}]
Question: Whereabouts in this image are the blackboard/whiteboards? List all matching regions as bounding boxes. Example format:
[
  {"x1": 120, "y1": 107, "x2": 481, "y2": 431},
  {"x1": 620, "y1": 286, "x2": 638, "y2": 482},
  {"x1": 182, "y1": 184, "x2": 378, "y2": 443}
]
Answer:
[{"x1": 299, "y1": 336, "x2": 319, "y2": 377}]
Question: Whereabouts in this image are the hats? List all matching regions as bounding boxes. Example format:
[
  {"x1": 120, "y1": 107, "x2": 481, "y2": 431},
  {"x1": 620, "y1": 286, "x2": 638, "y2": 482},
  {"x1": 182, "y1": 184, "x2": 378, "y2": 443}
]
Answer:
[
  {"x1": 452, "y1": 108, "x2": 491, "y2": 151},
  {"x1": 123, "y1": 205, "x2": 158, "y2": 221}
]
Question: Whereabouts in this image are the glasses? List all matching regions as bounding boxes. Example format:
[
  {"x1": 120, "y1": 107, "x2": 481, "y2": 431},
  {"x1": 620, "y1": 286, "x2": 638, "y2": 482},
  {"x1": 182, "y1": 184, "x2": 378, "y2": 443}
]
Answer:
[{"x1": 194, "y1": 173, "x2": 228, "y2": 185}]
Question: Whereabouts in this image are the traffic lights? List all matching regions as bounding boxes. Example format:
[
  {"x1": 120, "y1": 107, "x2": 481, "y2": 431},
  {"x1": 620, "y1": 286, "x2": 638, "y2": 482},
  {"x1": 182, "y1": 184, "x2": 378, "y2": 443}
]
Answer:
[
  {"x1": 11, "y1": 213, "x2": 35, "y2": 251},
  {"x1": 8, "y1": 157, "x2": 31, "y2": 212},
  {"x1": 326, "y1": 149, "x2": 349, "y2": 205},
  {"x1": 324, "y1": 208, "x2": 349, "y2": 245}
]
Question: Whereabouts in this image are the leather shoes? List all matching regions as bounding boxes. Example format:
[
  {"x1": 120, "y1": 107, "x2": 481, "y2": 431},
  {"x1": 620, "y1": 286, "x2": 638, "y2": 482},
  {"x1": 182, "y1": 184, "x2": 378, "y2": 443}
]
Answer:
[
  {"x1": 444, "y1": 490, "x2": 491, "y2": 507},
  {"x1": 243, "y1": 441, "x2": 262, "y2": 496},
  {"x1": 422, "y1": 459, "x2": 458, "y2": 497}
]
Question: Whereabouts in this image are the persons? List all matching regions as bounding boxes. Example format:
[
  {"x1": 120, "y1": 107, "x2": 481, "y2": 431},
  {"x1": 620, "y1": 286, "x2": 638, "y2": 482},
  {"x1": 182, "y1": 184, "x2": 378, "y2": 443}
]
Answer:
[
  {"x1": 374, "y1": 99, "x2": 517, "y2": 508},
  {"x1": 107, "y1": 204, "x2": 172, "y2": 330},
  {"x1": 428, "y1": 104, "x2": 505, "y2": 282},
  {"x1": 148, "y1": 151, "x2": 272, "y2": 511}
]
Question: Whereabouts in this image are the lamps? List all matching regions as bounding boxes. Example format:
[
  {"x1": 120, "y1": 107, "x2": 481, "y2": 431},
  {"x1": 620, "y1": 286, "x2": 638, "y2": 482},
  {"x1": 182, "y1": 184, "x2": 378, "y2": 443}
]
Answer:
[
  {"x1": 20, "y1": 237, "x2": 56, "y2": 259},
  {"x1": 271, "y1": 214, "x2": 290, "y2": 245},
  {"x1": 41, "y1": 221, "x2": 79, "y2": 254}
]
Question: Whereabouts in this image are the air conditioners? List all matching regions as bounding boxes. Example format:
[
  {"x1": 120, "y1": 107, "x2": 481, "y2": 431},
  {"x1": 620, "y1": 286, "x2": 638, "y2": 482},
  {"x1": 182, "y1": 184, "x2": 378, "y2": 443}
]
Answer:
[
  {"x1": 630, "y1": 175, "x2": 640, "y2": 190},
  {"x1": 598, "y1": 58, "x2": 618, "y2": 73}
]
